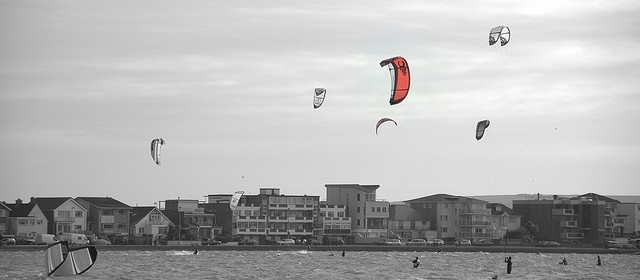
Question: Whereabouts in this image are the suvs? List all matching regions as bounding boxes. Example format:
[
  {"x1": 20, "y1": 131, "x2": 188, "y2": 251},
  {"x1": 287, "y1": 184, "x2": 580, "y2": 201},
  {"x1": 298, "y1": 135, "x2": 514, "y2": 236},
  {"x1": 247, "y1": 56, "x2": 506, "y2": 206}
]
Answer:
[{"x1": 25, "y1": 237, "x2": 36, "y2": 245}]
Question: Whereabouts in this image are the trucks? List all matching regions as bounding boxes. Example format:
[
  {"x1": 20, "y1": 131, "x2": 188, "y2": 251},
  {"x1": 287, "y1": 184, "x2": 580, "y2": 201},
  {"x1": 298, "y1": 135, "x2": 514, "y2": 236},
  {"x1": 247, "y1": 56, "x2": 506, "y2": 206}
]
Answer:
[
  {"x1": 36, "y1": 234, "x2": 59, "y2": 244},
  {"x1": 60, "y1": 233, "x2": 89, "y2": 245},
  {"x1": 201, "y1": 238, "x2": 221, "y2": 246}
]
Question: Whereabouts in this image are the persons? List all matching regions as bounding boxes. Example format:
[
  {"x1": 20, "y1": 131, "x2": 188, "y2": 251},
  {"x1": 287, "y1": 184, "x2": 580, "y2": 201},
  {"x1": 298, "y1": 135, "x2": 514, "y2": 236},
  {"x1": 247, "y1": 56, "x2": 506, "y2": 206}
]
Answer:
[
  {"x1": 413, "y1": 257, "x2": 421, "y2": 267},
  {"x1": 597, "y1": 256, "x2": 601, "y2": 266},
  {"x1": 505, "y1": 257, "x2": 513, "y2": 274},
  {"x1": 562, "y1": 258, "x2": 568, "y2": 266}
]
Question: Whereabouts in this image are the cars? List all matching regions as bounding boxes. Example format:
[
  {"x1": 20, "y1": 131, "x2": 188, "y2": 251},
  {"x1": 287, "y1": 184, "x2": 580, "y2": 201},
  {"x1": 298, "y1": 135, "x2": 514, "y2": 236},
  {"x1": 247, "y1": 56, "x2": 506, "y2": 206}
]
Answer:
[
  {"x1": 427, "y1": 239, "x2": 445, "y2": 246},
  {"x1": 277, "y1": 239, "x2": 295, "y2": 244},
  {"x1": 456, "y1": 239, "x2": 470, "y2": 246},
  {"x1": 473, "y1": 239, "x2": 494, "y2": 246},
  {"x1": 385, "y1": 239, "x2": 401, "y2": 245},
  {"x1": 407, "y1": 239, "x2": 427, "y2": 246},
  {"x1": 238, "y1": 239, "x2": 258, "y2": 245}
]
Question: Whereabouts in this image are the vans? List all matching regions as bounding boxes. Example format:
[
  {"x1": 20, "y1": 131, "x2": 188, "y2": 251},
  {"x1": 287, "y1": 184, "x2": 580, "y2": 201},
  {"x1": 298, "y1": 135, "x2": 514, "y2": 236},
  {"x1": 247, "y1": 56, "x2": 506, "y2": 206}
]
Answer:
[{"x1": 323, "y1": 237, "x2": 344, "y2": 245}]
